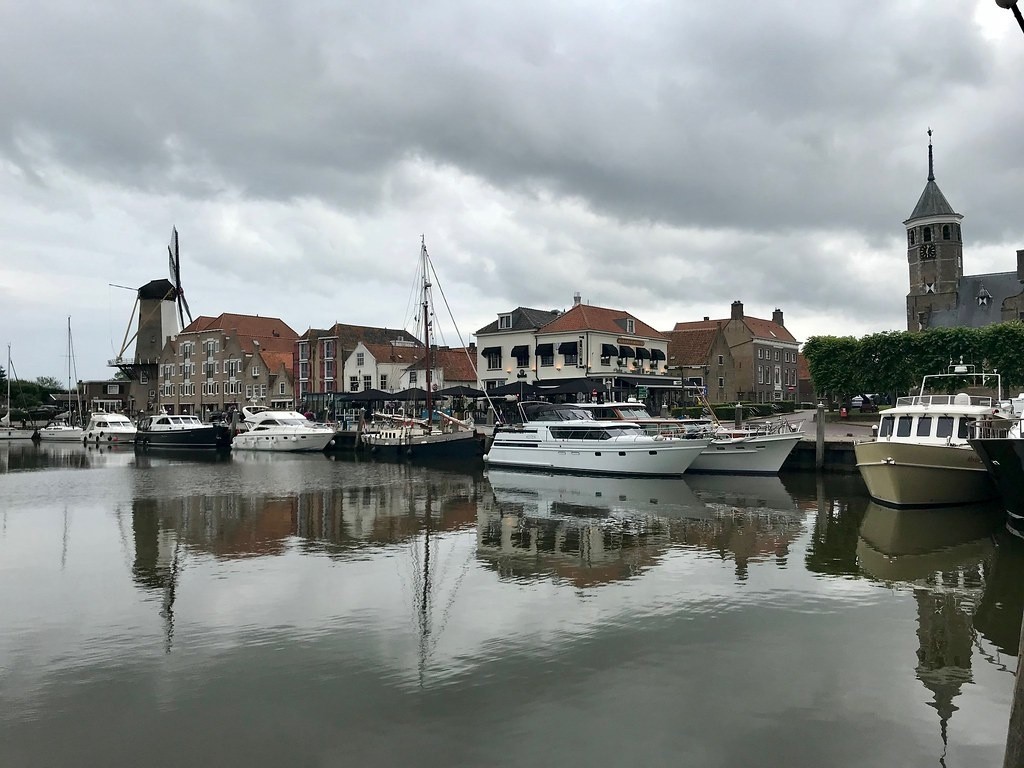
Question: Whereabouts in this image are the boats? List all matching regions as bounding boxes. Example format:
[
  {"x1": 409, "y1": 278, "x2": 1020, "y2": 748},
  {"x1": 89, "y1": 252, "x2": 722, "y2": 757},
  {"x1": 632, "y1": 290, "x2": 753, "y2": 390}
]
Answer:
[
  {"x1": 682, "y1": 470, "x2": 799, "y2": 514},
  {"x1": 526, "y1": 402, "x2": 808, "y2": 474},
  {"x1": 131, "y1": 414, "x2": 220, "y2": 449},
  {"x1": 967, "y1": 390, "x2": 1024, "y2": 492},
  {"x1": 994, "y1": 484, "x2": 1024, "y2": 548},
  {"x1": 483, "y1": 465, "x2": 717, "y2": 523},
  {"x1": 231, "y1": 403, "x2": 337, "y2": 453},
  {"x1": 482, "y1": 416, "x2": 715, "y2": 480},
  {"x1": 849, "y1": 354, "x2": 1010, "y2": 508},
  {"x1": 856, "y1": 496, "x2": 1004, "y2": 590}
]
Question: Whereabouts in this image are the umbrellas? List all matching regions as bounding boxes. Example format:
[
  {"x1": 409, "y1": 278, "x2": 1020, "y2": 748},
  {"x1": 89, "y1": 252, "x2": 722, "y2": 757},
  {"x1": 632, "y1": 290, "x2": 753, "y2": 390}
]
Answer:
[{"x1": 337, "y1": 379, "x2": 624, "y2": 422}]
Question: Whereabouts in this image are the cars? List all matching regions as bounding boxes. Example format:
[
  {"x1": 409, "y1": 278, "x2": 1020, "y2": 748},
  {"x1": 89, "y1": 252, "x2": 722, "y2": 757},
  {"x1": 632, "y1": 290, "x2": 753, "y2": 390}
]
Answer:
[
  {"x1": 9, "y1": 403, "x2": 77, "y2": 420},
  {"x1": 204, "y1": 410, "x2": 224, "y2": 421},
  {"x1": 78, "y1": 409, "x2": 137, "y2": 444},
  {"x1": 852, "y1": 393, "x2": 877, "y2": 409}
]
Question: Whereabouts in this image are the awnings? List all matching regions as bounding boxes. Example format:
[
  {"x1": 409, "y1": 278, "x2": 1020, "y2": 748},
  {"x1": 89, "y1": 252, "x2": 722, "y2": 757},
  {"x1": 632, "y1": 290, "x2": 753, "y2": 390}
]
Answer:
[
  {"x1": 618, "y1": 377, "x2": 706, "y2": 389},
  {"x1": 600, "y1": 344, "x2": 666, "y2": 361},
  {"x1": 481, "y1": 342, "x2": 579, "y2": 358}
]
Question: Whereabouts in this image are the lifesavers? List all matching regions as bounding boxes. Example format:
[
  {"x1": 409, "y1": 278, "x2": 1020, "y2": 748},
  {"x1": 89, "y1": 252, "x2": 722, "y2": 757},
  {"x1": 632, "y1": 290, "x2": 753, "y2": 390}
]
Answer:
[{"x1": 403, "y1": 418, "x2": 414, "y2": 429}]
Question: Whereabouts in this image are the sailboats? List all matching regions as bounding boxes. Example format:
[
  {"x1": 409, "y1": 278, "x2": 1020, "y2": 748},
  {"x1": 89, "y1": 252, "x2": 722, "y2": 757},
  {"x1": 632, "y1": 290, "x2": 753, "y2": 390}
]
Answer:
[
  {"x1": 357, "y1": 235, "x2": 490, "y2": 457},
  {"x1": 0, "y1": 341, "x2": 41, "y2": 440},
  {"x1": 34, "y1": 315, "x2": 84, "y2": 440}
]
